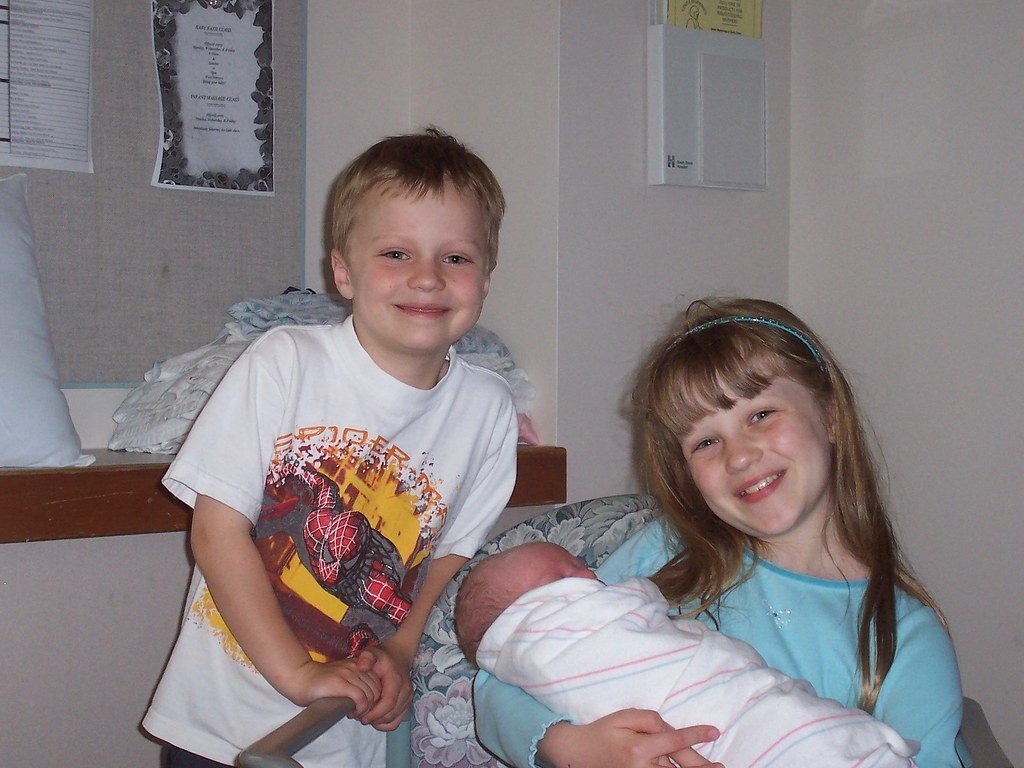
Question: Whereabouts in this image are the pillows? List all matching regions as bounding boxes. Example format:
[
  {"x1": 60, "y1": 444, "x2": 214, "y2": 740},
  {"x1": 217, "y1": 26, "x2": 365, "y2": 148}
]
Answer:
[{"x1": 0, "y1": 173, "x2": 99, "y2": 469}]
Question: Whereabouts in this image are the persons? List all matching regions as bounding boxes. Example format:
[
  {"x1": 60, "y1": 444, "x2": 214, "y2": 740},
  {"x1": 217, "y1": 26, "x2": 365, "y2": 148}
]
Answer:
[
  {"x1": 470, "y1": 295, "x2": 961, "y2": 768},
  {"x1": 455, "y1": 542, "x2": 913, "y2": 768},
  {"x1": 142, "y1": 126, "x2": 520, "y2": 768}
]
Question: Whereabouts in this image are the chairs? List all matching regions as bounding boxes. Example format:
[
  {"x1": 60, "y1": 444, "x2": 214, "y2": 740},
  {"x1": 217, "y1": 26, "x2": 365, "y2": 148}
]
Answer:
[{"x1": 230, "y1": 496, "x2": 1015, "y2": 768}]
